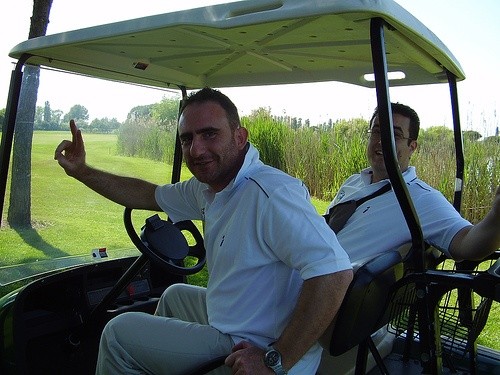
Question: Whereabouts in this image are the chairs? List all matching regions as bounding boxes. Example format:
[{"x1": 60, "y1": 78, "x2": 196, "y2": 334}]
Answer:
[{"x1": 314, "y1": 233, "x2": 448, "y2": 375}]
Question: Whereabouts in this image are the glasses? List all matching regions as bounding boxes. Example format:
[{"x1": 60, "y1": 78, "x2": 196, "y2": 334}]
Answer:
[{"x1": 367, "y1": 127, "x2": 412, "y2": 143}]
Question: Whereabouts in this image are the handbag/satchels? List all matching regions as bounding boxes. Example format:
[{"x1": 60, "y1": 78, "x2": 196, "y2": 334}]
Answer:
[{"x1": 327, "y1": 183, "x2": 393, "y2": 235}]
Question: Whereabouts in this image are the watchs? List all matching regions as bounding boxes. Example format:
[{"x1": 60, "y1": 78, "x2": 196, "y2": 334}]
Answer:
[{"x1": 264, "y1": 346, "x2": 288, "y2": 375}]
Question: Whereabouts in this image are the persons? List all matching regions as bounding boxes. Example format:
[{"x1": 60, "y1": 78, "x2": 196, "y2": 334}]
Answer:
[
  {"x1": 54, "y1": 84, "x2": 353, "y2": 375},
  {"x1": 318, "y1": 101, "x2": 500, "y2": 278}
]
